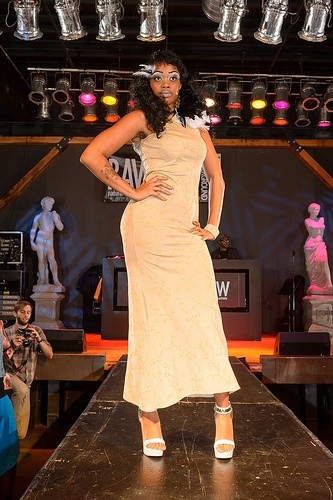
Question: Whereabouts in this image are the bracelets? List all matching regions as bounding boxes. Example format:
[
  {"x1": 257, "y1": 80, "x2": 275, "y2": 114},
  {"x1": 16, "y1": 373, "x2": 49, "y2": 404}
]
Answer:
[
  {"x1": 37, "y1": 340, "x2": 42, "y2": 343},
  {"x1": 204, "y1": 223, "x2": 220, "y2": 240}
]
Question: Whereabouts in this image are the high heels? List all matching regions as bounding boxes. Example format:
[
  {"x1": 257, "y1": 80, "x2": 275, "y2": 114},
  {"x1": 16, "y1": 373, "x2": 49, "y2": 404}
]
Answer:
[
  {"x1": 138, "y1": 407, "x2": 166, "y2": 456},
  {"x1": 213, "y1": 400, "x2": 235, "y2": 459}
]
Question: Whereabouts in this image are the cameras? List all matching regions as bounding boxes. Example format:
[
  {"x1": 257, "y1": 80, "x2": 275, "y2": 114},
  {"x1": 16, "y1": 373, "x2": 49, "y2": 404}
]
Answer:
[{"x1": 16, "y1": 328, "x2": 34, "y2": 347}]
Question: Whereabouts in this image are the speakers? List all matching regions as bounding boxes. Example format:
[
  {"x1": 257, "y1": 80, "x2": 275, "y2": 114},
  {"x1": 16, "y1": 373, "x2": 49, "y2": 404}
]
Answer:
[
  {"x1": 41, "y1": 329, "x2": 84, "y2": 353},
  {"x1": 274, "y1": 331, "x2": 331, "y2": 357}
]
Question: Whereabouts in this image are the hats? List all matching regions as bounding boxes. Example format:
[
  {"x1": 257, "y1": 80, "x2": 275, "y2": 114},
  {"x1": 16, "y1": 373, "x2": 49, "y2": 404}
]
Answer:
[{"x1": 215, "y1": 233, "x2": 231, "y2": 243}]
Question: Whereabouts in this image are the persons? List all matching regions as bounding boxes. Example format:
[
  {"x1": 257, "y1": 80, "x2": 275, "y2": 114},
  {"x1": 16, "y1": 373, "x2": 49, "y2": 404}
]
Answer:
[
  {"x1": 0, "y1": 301, "x2": 53, "y2": 500},
  {"x1": 80, "y1": 51, "x2": 241, "y2": 458},
  {"x1": 305, "y1": 203, "x2": 333, "y2": 294},
  {"x1": 209, "y1": 234, "x2": 242, "y2": 261},
  {"x1": 31, "y1": 197, "x2": 64, "y2": 286}
]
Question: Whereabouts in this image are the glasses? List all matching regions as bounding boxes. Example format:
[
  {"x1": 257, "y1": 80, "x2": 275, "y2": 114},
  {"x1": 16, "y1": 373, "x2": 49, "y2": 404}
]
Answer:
[{"x1": 220, "y1": 239, "x2": 230, "y2": 242}]
